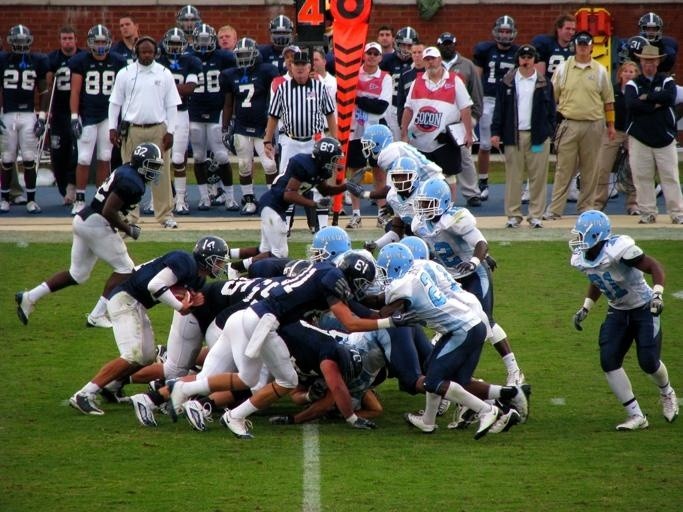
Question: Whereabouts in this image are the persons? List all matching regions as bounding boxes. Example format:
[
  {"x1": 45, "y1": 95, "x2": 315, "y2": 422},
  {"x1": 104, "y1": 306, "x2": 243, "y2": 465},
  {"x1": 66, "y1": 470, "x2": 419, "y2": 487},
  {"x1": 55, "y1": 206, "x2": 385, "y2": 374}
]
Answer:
[
  {"x1": 567, "y1": 209, "x2": 681, "y2": 433},
  {"x1": 0, "y1": 0, "x2": 683, "y2": 233},
  {"x1": 63, "y1": 232, "x2": 535, "y2": 446},
  {"x1": 14, "y1": 141, "x2": 165, "y2": 330}
]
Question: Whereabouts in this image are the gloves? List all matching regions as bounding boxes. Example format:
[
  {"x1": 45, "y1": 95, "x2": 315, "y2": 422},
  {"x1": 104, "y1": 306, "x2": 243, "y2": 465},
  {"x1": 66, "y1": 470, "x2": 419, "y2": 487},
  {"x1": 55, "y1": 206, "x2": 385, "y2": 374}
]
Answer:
[
  {"x1": 572, "y1": 306, "x2": 589, "y2": 332},
  {"x1": 650, "y1": 290, "x2": 664, "y2": 318},
  {"x1": 128, "y1": 222, "x2": 141, "y2": 241},
  {"x1": 483, "y1": 255, "x2": 498, "y2": 273},
  {"x1": 307, "y1": 378, "x2": 328, "y2": 403},
  {"x1": 350, "y1": 417, "x2": 379, "y2": 431},
  {"x1": 454, "y1": 261, "x2": 476, "y2": 275},
  {"x1": 269, "y1": 415, "x2": 289, "y2": 425},
  {"x1": 387, "y1": 304, "x2": 423, "y2": 330}
]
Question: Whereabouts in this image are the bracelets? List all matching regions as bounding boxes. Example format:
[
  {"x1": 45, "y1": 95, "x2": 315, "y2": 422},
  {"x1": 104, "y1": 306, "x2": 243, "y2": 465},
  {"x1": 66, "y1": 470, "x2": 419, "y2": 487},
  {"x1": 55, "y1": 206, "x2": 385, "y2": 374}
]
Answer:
[
  {"x1": 582, "y1": 297, "x2": 595, "y2": 312},
  {"x1": 651, "y1": 283, "x2": 665, "y2": 295}
]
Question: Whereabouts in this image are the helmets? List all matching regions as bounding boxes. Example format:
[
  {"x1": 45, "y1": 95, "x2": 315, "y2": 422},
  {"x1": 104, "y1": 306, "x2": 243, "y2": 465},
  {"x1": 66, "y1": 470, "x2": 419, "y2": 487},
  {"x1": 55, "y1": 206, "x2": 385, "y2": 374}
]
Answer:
[
  {"x1": 312, "y1": 225, "x2": 351, "y2": 264},
  {"x1": 9, "y1": 26, "x2": 33, "y2": 53},
  {"x1": 575, "y1": 209, "x2": 612, "y2": 250},
  {"x1": 399, "y1": 234, "x2": 431, "y2": 262},
  {"x1": 88, "y1": 25, "x2": 112, "y2": 53},
  {"x1": 347, "y1": 346, "x2": 363, "y2": 380},
  {"x1": 391, "y1": 156, "x2": 420, "y2": 192},
  {"x1": 362, "y1": 123, "x2": 394, "y2": 154},
  {"x1": 377, "y1": 242, "x2": 414, "y2": 283},
  {"x1": 161, "y1": 4, "x2": 666, "y2": 64},
  {"x1": 130, "y1": 141, "x2": 163, "y2": 175},
  {"x1": 191, "y1": 234, "x2": 230, "y2": 270},
  {"x1": 339, "y1": 252, "x2": 376, "y2": 284},
  {"x1": 313, "y1": 137, "x2": 344, "y2": 167},
  {"x1": 417, "y1": 177, "x2": 452, "y2": 213}
]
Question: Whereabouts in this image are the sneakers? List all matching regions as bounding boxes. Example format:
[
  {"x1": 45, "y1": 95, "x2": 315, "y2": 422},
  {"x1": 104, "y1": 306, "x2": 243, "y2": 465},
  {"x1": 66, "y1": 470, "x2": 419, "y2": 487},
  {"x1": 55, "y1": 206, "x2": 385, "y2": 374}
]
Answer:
[
  {"x1": 155, "y1": 344, "x2": 169, "y2": 367},
  {"x1": 224, "y1": 263, "x2": 242, "y2": 282},
  {"x1": 658, "y1": 385, "x2": 680, "y2": 425},
  {"x1": 401, "y1": 411, "x2": 439, "y2": 435},
  {"x1": 181, "y1": 399, "x2": 210, "y2": 433},
  {"x1": 67, "y1": 389, "x2": 105, "y2": 417},
  {"x1": 217, "y1": 406, "x2": 255, "y2": 440},
  {"x1": 0, "y1": 179, "x2": 683, "y2": 230},
  {"x1": 85, "y1": 313, "x2": 114, "y2": 329},
  {"x1": 148, "y1": 377, "x2": 170, "y2": 416},
  {"x1": 166, "y1": 379, "x2": 188, "y2": 425},
  {"x1": 435, "y1": 367, "x2": 531, "y2": 440},
  {"x1": 99, "y1": 386, "x2": 132, "y2": 404},
  {"x1": 616, "y1": 414, "x2": 650, "y2": 431},
  {"x1": 14, "y1": 290, "x2": 34, "y2": 326},
  {"x1": 129, "y1": 391, "x2": 158, "y2": 430}
]
